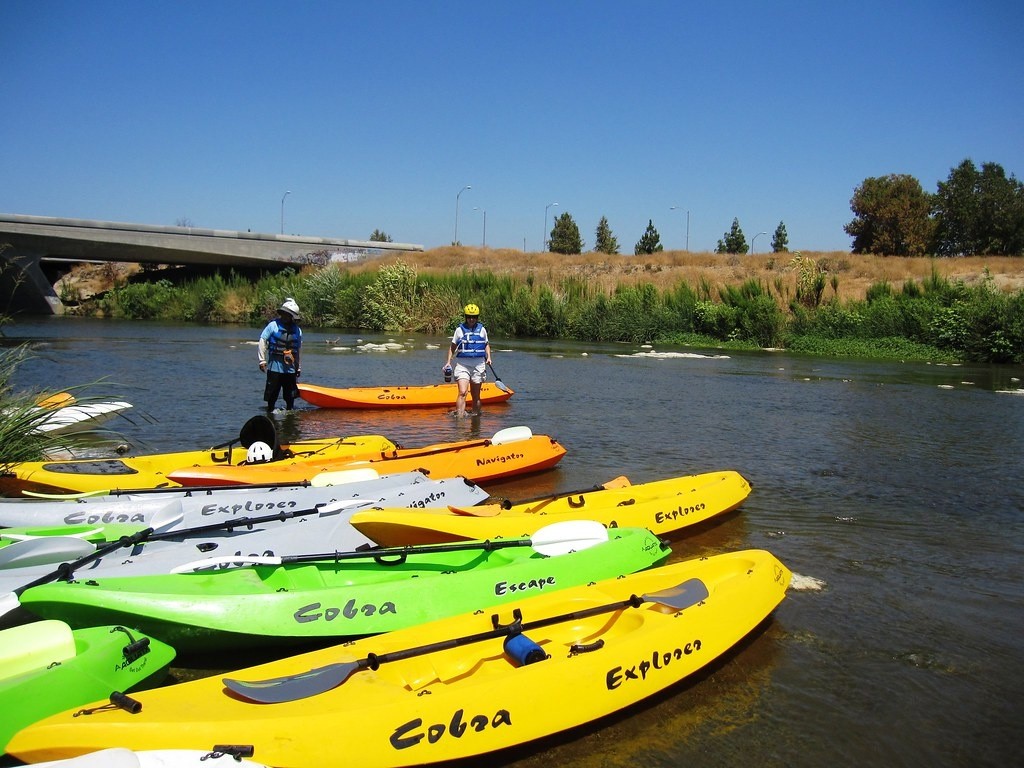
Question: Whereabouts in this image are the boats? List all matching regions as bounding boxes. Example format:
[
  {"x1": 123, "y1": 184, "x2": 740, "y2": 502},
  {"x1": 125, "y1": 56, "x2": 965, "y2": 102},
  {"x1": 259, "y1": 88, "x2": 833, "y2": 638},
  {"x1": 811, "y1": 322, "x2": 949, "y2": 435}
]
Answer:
[
  {"x1": 3, "y1": 550, "x2": 793, "y2": 768},
  {"x1": 0, "y1": 435, "x2": 567, "y2": 498},
  {"x1": 296, "y1": 383, "x2": 514, "y2": 410},
  {"x1": 0, "y1": 471, "x2": 752, "y2": 758},
  {"x1": 0, "y1": 402, "x2": 133, "y2": 436}
]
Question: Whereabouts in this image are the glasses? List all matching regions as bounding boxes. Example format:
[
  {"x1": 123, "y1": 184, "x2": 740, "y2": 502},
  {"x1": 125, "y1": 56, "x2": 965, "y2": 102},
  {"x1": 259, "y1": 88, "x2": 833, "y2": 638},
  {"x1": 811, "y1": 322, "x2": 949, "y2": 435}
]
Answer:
[{"x1": 467, "y1": 315, "x2": 479, "y2": 319}]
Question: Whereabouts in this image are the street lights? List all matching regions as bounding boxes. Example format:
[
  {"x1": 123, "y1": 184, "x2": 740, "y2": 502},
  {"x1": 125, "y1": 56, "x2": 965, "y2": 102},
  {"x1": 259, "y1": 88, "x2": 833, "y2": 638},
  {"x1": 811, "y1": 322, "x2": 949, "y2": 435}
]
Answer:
[
  {"x1": 455, "y1": 185, "x2": 471, "y2": 246},
  {"x1": 752, "y1": 232, "x2": 767, "y2": 254},
  {"x1": 282, "y1": 191, "x2": 291, "y2": 234},
  {"x1": 544, "y1": 202, "x2": 559, "y2": 253},
  {"x1": 670, "y1": 207, "x2": 689, "y2": 250},
  {"x1": 473, "y1": 208, "x2": 485, "y2": 245}
]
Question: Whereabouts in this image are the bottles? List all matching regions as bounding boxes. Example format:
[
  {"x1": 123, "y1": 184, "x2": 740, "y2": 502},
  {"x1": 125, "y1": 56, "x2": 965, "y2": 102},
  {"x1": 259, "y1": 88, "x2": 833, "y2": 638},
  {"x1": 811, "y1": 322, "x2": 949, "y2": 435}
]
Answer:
[
  {"x1": 445, "y1": 365, "x2": 452, "y2": 382},
  {"x1": 283, "y1": 349, "x2": 294, "y2": 365}
]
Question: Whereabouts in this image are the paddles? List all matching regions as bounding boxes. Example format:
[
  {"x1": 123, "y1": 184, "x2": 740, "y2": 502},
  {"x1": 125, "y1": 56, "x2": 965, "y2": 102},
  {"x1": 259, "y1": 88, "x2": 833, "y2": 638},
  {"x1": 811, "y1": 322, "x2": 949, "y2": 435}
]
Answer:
[
  {"x1": 0, "y1": 494, "x2": 186, "y2": 617},
  {"x1": 192, "y1": 520, "x2": 609, "y2": 570},
  {"x1": 447, "y1": 475, "x2": 631, "y2": 518},
  {"x1": 487, "y1": 362, "x2": 511, "y2": 396},
  {"x1": 223, "y1": 577, "x2": 711, "y2": 705},
  {"x1": 0, "y1": 499, "x2": 379, "y2": 570},
  {"x1": 0, "y1": 618, "x2": 77, "y2": 681},
  {"x1": 21, "y1": 468, "x2": 379, "y2": 499}
]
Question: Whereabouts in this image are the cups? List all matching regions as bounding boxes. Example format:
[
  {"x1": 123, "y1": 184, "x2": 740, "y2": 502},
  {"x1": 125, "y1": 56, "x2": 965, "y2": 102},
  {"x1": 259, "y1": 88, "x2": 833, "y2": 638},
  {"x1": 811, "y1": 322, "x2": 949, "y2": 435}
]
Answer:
[{"x1": 503, "y1": 633, "x2": 546, "y2": 667}]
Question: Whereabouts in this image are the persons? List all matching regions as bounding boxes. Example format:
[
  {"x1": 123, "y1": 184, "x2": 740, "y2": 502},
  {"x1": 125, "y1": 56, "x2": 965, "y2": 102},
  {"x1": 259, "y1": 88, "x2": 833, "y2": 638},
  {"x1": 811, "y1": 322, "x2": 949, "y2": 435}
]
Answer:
[
  {"x1": 257, "y1": 301, "x2": 303, "y2": 411},
  {"x1": 441, "y1": 303, "x2": 492, "y2": 415}
]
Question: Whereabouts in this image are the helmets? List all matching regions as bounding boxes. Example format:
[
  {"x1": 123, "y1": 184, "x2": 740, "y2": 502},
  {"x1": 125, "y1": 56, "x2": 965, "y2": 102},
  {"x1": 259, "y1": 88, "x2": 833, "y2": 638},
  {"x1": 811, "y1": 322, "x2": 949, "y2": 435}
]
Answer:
[
  {"x1": 277, "y1": 297, "x2": 302, "y2": 322},
  {"x1": 247, "y1": 441, "x2": 272, "y2": 463},
  {"x1": 463, "y1": 303, "x2": 480, "y2": 316}
]
